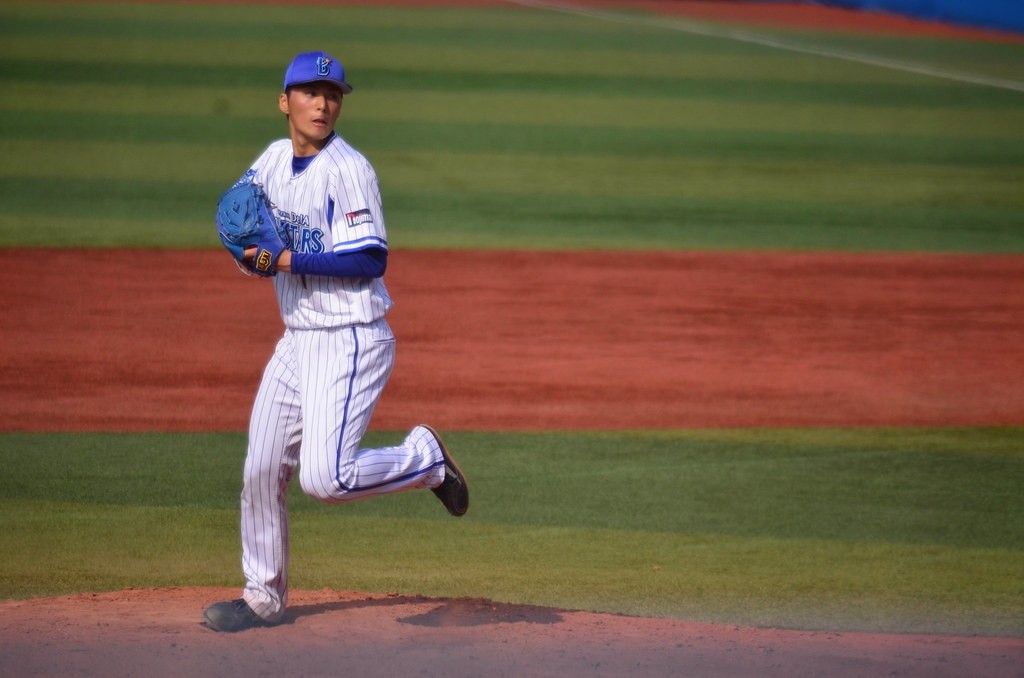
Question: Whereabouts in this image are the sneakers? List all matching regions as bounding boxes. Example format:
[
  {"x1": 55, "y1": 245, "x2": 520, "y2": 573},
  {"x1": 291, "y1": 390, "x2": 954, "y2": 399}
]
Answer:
[
  {"x1": 419, "y1": 424, "x2": 470, "y2": 517},
  {"x1": 203, "y1": 599, "x2": 271, "y2": 632}
]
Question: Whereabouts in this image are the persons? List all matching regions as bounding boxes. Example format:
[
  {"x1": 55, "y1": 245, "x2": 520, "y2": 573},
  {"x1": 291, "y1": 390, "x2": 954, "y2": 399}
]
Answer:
[{"x1": 204, "y1": 51, "x2": 469, "y2": 632}]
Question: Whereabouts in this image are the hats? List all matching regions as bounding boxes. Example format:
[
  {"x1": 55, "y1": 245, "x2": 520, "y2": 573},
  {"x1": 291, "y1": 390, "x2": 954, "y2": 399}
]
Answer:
[{"x1": 285, "y1": 52, "x2": 354, "y2": 95}]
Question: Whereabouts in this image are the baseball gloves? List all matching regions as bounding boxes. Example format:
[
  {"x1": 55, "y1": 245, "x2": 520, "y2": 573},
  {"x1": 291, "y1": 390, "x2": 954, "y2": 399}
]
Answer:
[{"x1": 213, "y1": 180, "x2": 292, "y2": 277}]
